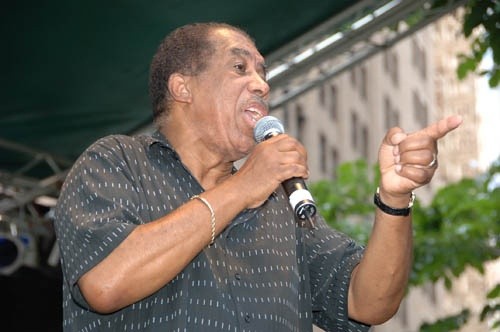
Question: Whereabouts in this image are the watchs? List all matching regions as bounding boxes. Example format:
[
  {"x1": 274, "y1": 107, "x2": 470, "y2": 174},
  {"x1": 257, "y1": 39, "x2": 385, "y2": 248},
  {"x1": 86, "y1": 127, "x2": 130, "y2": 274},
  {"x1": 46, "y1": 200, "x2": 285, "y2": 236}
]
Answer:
[{"x1": 374, "y1": 187, "x2": 416, "y2": 217}]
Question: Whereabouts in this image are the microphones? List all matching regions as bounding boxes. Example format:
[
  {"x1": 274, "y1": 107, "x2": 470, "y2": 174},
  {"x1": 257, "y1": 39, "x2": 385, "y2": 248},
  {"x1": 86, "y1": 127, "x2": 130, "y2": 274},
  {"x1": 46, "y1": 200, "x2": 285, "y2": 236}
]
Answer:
[{"x1": 254, "y1": 115, "x2": 318, "y2": 230}]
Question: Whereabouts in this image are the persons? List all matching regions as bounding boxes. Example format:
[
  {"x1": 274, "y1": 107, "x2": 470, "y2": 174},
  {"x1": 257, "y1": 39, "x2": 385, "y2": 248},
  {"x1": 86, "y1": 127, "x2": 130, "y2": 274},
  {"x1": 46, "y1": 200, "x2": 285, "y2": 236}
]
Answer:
[{"x1": 55, "y1": 24, "x2": 462, "y2": 332}]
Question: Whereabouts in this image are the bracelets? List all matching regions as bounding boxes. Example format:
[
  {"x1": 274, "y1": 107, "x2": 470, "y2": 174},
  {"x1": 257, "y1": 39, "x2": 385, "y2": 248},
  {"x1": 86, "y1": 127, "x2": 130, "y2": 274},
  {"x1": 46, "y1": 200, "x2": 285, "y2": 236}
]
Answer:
[{"x1": 190, "y1": 195, "x2": 216, "y2": 245}]
